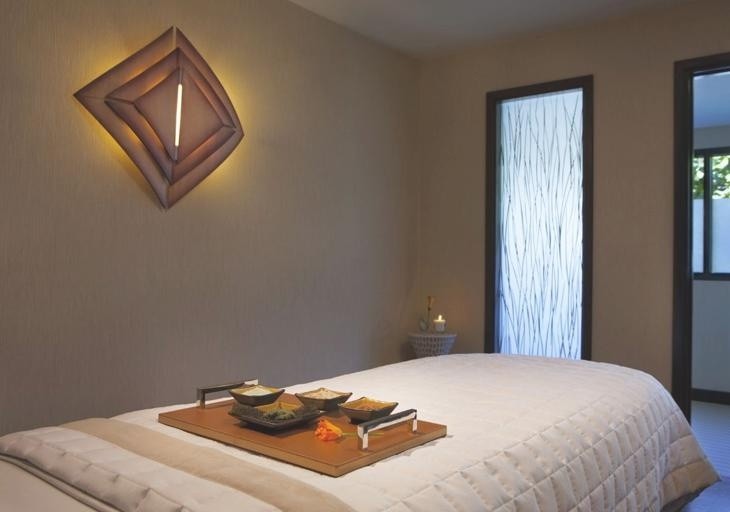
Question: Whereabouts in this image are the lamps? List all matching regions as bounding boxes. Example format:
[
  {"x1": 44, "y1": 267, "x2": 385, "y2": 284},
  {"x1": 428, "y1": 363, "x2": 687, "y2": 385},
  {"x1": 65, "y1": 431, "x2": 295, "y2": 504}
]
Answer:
[{"x1": 69, "y1": 24, "x2": 245, "y2": 209}]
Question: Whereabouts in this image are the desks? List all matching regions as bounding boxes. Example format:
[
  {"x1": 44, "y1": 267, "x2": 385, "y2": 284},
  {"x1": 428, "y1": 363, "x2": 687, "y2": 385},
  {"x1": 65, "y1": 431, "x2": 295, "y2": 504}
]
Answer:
[{"x1": 408, "y1": 332, "x2": 458, "y2": 359}]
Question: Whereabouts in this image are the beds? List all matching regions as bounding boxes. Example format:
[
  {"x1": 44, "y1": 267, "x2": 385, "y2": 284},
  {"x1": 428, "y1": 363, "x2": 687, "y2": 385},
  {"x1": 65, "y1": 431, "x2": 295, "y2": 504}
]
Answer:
[{"x1": 0, "y1": 352, "x2": 722, "y2": 510}]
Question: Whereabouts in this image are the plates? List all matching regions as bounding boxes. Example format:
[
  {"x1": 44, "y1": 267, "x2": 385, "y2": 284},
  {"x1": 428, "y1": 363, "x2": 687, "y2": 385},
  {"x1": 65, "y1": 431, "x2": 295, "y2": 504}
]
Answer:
[{"x1": 228, "y1": 401, "x2": 328, "y2": 431}]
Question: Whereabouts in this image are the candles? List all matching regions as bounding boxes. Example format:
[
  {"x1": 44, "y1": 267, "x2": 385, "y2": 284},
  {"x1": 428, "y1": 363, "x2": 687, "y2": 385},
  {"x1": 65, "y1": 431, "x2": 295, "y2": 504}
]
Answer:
[{"x1": 433, "y1": 314, "x2": 446, "y2": 333}]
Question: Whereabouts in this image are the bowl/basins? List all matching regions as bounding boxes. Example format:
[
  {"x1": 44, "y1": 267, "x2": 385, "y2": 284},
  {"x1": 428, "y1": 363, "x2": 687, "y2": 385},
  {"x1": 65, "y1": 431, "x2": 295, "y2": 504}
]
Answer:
[{"x1": 227, "y1": 383, "x2": 398, "y2": 424}]
